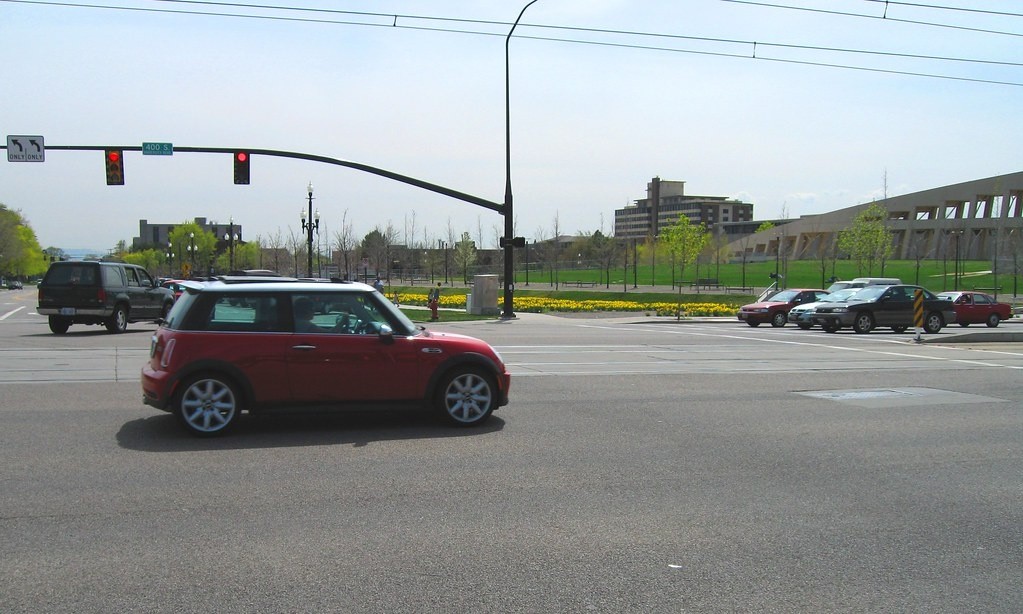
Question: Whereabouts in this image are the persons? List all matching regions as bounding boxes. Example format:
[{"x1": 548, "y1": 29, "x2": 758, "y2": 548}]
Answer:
[
  {"x1": 961, "y1": 298, "x2": 967, "y2": 303},
  {"x1": 434, "y1": 282, "x2": 441, "y2": 308},
  {"x1": 393, "y1": 291, "x2": 399, "y2": 307},
  {"x1": 293, "y1": 298, "x2": 348, "y2": 333},
  {"x1": 374, "y1": 276, "x2": 385, "y2": 294},
  {"x1": 427, "y1": 288, "x2": 435, "y2": 308}
]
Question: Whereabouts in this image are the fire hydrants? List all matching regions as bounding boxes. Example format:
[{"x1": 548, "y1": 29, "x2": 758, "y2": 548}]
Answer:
[{"x1": 429, "y1": 296, "x2": 439, "y2": 318}]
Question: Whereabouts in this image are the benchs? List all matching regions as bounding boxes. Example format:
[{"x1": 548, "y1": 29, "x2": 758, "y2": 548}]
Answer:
[
  {"x1": 358, "y1": 274, "x2": 377, "y2": 282},
  {"x1": 405, "y1": 279, "x2": 430, "y2": 283},
  {"x1": 674, "y1": 278, "x2": 724, "y2": 291},
  {"x1": 466, "y1": 282, "x2": 474, "y2": 287},
  {"x1": 725, "y1": 285, "x2": 753, "y2": 295},
  {"x1": 562, "y1": 280, "x2": 597, "y2": 288},
  {"x1": 972, "y1": 285, "x2": 1003, "y2": 294}
]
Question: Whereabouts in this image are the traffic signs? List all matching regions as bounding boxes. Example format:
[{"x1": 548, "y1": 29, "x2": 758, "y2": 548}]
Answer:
[{"x1": 6, "y1": 134, "x2": 45, "y2": 162}]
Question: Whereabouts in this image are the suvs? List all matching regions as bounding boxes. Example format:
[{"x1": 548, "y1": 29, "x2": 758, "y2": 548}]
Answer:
[
  {"x1": 141, "y1": 270, "x2": 510, "y2": 438},
  {"x1": 36, "y1": 260, "x2": 175, "y2": 335}
]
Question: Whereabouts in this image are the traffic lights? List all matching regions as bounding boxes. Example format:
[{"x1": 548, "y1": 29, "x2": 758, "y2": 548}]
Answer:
[
  {"x1": 103, "y1": 148, "x2": 126, "y2": 186},
  {"x1": 234, "y1": 152, "x2": 250, "y2": 185}
]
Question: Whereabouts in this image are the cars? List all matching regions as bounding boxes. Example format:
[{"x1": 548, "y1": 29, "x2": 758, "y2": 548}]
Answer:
[
  {"x1": 157, "y1": 277, "x2": 187, "y2": 301},
  {"x1": 216, "y1": 295, "x2": 372, "y2": 316},
  {"x1": 936, "y1": 292, "x2": 1014, "y2": 328},
  {"x1": 9, "y1": 281, "x2": 23, "y2": 289},
  {"x1": 736, "y1": 289, "x2": 832, "y2": 328},
  {"x1": 787, "y1": 287, "x2": 863, "y2": 330},
  {"x1": 811, "y1": 284, "x2": 957, "y2": 335},
  {"x1": 36, "y1": 279, "x2": 43, "y2": 288}
]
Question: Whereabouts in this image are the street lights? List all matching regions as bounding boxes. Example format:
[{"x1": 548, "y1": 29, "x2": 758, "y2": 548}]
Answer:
[
  {"x1": 299, "y1": 181, "x2": 320, "y2": 278},
  {"x1": 225, "y1": 218, "x2": 238, "y2": 276},
  {"x1": 165, "y1": 242, "x2": 175, "y2": 277},
  {"x1": 950, "y1": 230, "x2": 963, "y2": 292},
  {"x1": 186, "y1": 231, "x2": 198, "y2": 280}
]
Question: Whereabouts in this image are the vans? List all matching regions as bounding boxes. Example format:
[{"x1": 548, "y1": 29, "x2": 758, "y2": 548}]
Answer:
[{"x1": 828, "y1": 277, "x2": 905, "y2": 294}]
